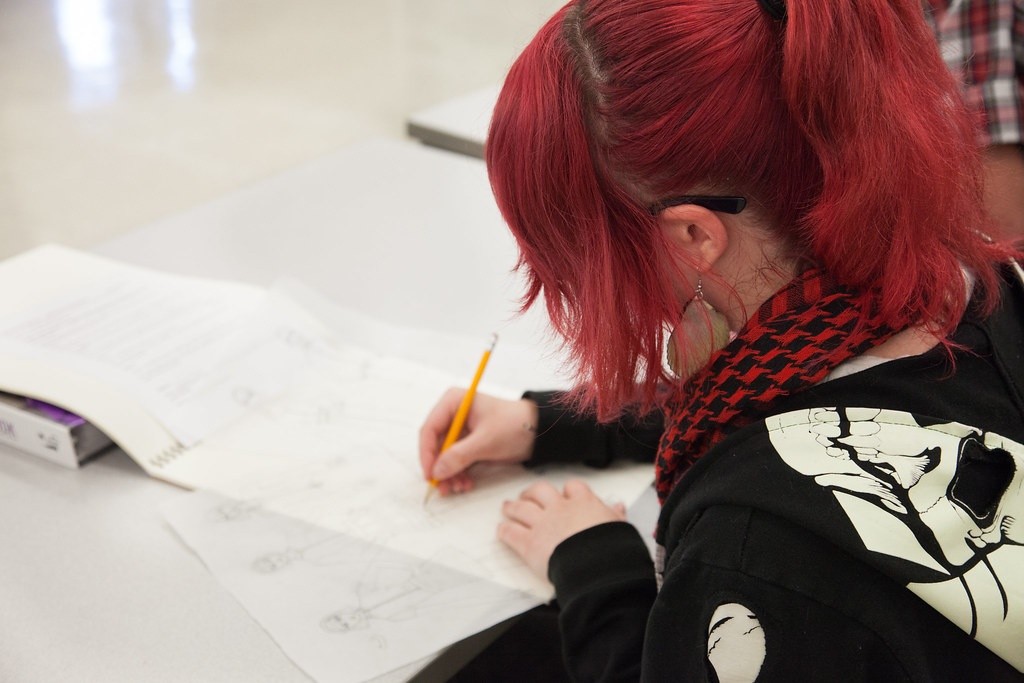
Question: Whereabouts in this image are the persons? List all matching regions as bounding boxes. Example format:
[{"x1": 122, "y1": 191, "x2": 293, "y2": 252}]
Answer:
[
  {"x1": 414, "y1": 0, "x2": 1024, "y2": 683},
  {"x1": 923, "y1": 1, "x2": 1024, "y2": 272}
]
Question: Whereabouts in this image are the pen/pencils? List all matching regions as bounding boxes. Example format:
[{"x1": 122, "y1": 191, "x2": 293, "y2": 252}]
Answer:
[{"x1": 420, "y1": 332, "x2": 500, "y2": 515}]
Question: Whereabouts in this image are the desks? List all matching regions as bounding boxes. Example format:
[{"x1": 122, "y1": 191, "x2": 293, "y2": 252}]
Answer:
[
  {"x1": 405, "y1": 78, "x2": 507, "y2": 156},
  {"x1": 0, "y1": 135, "x2": 661, "y2": 683}
]
